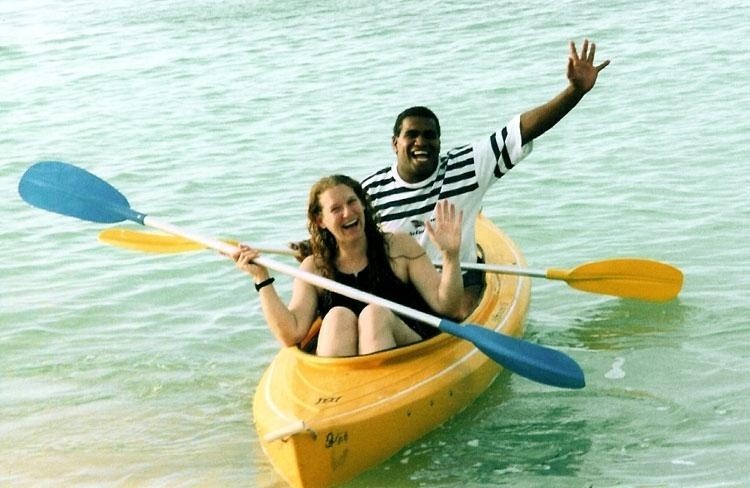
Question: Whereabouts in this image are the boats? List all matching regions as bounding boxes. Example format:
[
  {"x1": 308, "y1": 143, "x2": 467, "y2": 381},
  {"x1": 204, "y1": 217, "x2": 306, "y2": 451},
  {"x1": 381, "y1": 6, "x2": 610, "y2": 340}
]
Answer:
[{"x1": 252, "y1": 202, "x2": 531, "y2": 486}]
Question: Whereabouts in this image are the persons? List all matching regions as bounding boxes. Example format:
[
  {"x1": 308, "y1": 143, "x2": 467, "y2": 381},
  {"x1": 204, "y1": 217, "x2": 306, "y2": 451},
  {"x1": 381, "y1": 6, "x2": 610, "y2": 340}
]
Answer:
[
  {"x1": 235, "y1": 173, "x2": 467, "y2": 356},
  {"x1": 287, "y1": 39, "x2": 611, "y2": 321}
]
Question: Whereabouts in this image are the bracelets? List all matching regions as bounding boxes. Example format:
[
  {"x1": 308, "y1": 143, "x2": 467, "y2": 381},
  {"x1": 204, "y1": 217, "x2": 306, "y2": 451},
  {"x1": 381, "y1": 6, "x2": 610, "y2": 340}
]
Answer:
[{"x1": 253, "y1": 276, "x2": 276, "y2": 292}]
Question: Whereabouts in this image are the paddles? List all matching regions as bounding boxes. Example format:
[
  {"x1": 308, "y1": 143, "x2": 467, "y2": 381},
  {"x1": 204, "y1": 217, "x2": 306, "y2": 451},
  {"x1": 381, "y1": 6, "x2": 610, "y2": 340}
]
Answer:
[
  {"x1": 19, "y1": 161, "x2": 585, "y2": 388},
  {"x1": 99, "y1": 228, "x2": 683, "y2": 301}
]
